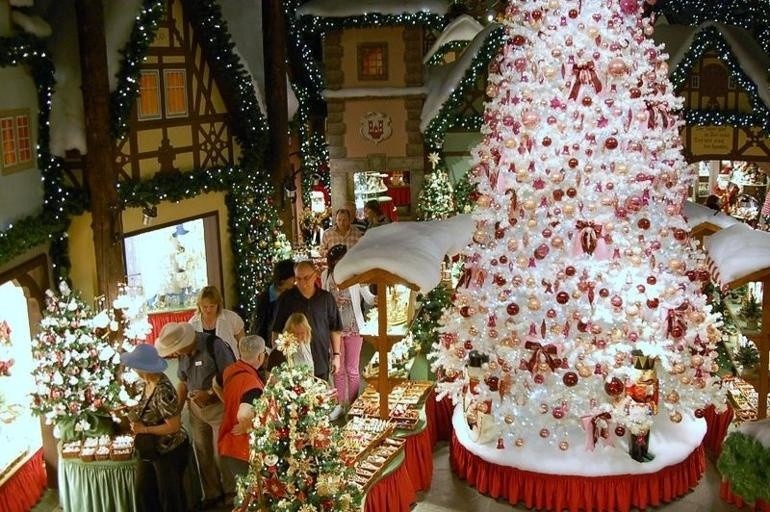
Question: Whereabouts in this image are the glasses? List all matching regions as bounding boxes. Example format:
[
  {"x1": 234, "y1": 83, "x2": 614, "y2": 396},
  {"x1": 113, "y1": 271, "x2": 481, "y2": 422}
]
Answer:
[{"x1": 294, "y1": 269, "x2": 316, "y2": 282}]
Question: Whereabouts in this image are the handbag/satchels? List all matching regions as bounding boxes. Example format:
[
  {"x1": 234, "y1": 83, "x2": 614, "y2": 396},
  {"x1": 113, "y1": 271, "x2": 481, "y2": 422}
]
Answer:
[{"x1": 133, "y1": 432, "x2": 164, "y2": 462}]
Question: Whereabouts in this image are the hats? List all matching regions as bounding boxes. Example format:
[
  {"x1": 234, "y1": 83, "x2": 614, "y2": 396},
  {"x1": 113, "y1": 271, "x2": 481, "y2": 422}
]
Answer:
[
  {"x1": 273, "y1": 259, "x2": 296, "y2": 281},
  {"x1": 154, "y1": 321, "x2": 195, "y2": 358},
  {"x1": 120, "y1": 343, "x2": 168, "y2": 373}
]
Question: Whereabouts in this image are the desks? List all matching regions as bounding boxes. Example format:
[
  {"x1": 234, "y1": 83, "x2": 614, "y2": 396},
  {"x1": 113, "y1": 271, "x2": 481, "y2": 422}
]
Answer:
[
  {"x1": 344, "y1": 380, "x2": 437, "y2": 511},
  {"x1": 56, "y1": 431, "x2": 199, "y2": 511}
]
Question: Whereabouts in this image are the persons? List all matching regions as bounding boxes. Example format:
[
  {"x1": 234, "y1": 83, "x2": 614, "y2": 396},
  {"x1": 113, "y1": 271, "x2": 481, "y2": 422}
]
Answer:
[
  {"x1": 712, "y1": 167, "x2": 740, "y2": 216},
  {"x1": 704, "y1": 194, "x2": 721, "y2": 211},
  {"x1": 251, "y1": 201, "x2": 388, "y2": 422},
  {"x1": 112, "y1": 285, "x2": 315, "y2": 512}
]
